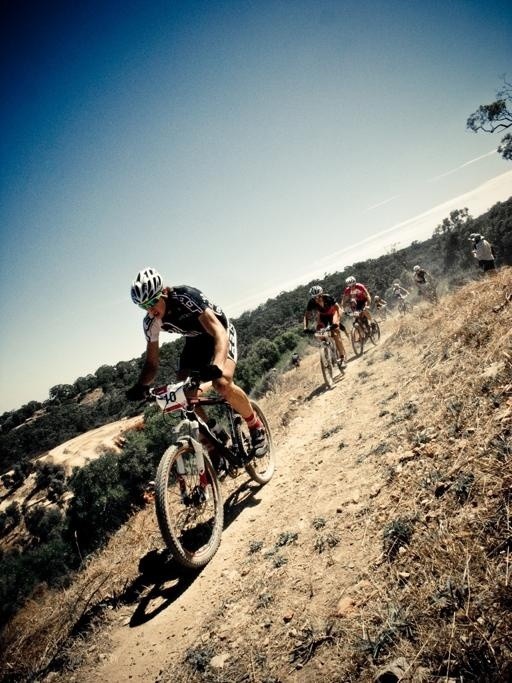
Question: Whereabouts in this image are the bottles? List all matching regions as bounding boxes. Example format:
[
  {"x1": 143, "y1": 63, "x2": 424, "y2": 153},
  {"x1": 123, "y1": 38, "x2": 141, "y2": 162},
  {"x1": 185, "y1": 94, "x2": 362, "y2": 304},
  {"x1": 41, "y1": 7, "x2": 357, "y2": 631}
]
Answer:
[{"x1": 204, "y1": 416, "x2": 231, "y2": 445}]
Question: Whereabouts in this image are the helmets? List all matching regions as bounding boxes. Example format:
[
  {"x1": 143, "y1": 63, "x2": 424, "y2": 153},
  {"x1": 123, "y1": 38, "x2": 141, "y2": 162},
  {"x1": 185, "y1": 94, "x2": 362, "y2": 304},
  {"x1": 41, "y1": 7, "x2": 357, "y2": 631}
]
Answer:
[
  {"x1": 345, "y1": 277, "x2": 357, "y2": 287},
  {"x1": 309, "y1": 286, "x2": 323, "y2": 298},
  {"x1": 132, "y1": 267, "x2": 163, "y2": 306}
]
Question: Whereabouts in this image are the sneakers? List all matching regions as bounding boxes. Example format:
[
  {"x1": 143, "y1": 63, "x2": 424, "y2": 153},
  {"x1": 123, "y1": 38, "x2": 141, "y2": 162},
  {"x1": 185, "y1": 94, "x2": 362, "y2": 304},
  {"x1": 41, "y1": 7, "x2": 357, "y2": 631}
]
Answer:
[
  {"x1": 340, "y1": 359, "x2": 347, "y2": 368},
  {"x1": 249, "y1": 427, "x2": 268, "y2": 457}
]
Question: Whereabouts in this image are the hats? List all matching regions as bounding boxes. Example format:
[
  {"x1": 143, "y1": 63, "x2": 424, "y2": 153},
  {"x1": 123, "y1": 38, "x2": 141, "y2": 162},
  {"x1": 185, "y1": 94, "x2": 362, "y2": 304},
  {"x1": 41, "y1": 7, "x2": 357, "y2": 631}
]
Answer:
[{"x1": 468, "y1": 233, "x2": 485, "y2": 242}]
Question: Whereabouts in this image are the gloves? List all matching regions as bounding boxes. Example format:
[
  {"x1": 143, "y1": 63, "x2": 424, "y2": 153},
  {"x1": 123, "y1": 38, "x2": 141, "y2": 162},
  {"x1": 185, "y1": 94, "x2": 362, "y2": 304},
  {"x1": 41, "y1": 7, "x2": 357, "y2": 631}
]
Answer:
[
  {"x1": 126, "y1": 384, "x2": 149, "y2": 401},
  {"x1": 198, "y1": 364, "x2": 222, "y2": 382},
  {"x1": 304, "y1": 329, "x2": 309, "y2": 333},
  {"x1": 330, "y1": 324, "x2": 338, "y2": 330}
]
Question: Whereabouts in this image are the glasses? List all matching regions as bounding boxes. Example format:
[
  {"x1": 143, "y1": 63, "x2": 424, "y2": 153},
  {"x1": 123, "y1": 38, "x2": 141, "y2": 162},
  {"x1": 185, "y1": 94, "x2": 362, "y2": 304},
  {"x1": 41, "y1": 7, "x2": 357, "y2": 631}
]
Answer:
[{"x1": 138, "y1": 292, "x2": 163, "y2": 310}]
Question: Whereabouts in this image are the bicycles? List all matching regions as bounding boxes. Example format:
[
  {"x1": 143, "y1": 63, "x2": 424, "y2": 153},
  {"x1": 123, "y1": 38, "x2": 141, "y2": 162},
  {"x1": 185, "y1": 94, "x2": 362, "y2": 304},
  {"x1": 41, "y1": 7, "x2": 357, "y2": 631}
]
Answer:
[
  {"x1": 374, "y1": 275, "x2": 439, "y2": 321},
  {"x1": 343, "y1": 305, "x2": 381, "y2": 357},
  {"x1": 131, "y1": 370, "x2": 274, "y2": 568},
  {"x1": 305, "y1": 324, "x2": 347, "y2": 388}
]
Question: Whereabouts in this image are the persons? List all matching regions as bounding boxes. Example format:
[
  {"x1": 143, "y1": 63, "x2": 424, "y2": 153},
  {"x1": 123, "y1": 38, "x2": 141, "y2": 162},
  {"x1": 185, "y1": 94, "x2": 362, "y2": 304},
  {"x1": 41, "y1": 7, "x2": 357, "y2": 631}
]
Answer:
[
  {"x1": 125, "y1": 267, "x2": 269, "y2": 484},
  {"x1": 303, "y1": 284, "x2": 348, "y2": 368},
  {"x1": 374, "y1": 232, "x2": 498, "y2": 322},
  {"x1": 341, "y1": 275, "x2": 372, "y2": 342}
]
